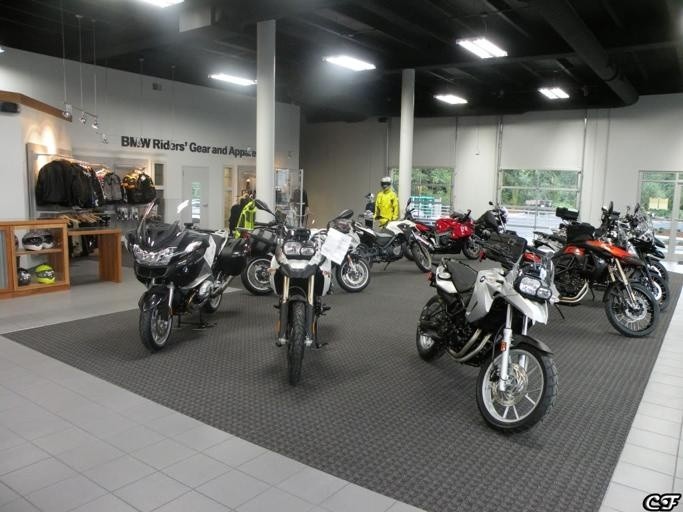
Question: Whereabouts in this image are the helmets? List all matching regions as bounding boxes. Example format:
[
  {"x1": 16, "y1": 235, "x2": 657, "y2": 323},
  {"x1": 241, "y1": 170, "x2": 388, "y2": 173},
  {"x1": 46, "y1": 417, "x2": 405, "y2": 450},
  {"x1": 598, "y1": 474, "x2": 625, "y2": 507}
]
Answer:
[
  {"x1": 17, "y1": 264, "x2": 56, "y2": 287},
  {"x1": 381, "y1": 177, "x2": 391, "y2": 183},
  {"x1": 15, "y1": 230, "x2": 54, "y2": 251}
]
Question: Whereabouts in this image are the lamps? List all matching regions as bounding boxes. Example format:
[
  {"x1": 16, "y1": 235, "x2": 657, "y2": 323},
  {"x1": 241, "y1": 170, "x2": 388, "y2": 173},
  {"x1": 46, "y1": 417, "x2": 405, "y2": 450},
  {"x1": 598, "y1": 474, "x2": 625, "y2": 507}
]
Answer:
[
  {"x1": 57, "y1": 8, "x2": 99, "y2": 131},
  {"x1": 457, "y1": 35, "x2": 510, "y2": 60},
  {"x1": 209, "y1": 68, "x2": 256, "y2": 87},
  {"x1": 324, "y1": 52, "x2": 376, "y2": 72},
  {"x1": 139, "y1": 1, "x2": 190, "y2": 10},
  {"x1": 536, "y1": 84, "x2": 571, "y2": 102}
]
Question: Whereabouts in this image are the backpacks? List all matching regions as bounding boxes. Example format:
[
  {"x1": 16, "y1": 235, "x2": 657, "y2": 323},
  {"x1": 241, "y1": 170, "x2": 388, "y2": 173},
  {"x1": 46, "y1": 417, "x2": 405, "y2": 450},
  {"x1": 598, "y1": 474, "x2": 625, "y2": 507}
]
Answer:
[{"x1": 122, "y1": 169, "x2": 156, "y2": 204}]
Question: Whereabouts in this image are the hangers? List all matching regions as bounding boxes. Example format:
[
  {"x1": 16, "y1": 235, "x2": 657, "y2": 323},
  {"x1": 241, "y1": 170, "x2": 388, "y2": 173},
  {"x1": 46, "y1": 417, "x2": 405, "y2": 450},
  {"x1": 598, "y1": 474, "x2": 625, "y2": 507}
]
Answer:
[{"x1": 57, "y1": 212, "x2": 96, "y2": 225}]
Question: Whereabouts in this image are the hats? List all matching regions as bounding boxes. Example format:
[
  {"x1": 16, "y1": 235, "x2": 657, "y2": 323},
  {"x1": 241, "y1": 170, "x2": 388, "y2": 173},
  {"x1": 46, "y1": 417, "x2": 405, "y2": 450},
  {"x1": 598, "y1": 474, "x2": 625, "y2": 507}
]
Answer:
[{"x1": 368, "y1": 193, "x2": 374, "y2": 197}]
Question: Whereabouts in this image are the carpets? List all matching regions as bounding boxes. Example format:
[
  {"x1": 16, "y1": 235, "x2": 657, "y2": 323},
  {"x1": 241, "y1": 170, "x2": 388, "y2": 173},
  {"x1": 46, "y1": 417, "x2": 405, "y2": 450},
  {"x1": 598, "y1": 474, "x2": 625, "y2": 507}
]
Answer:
[{"x1": 0, "y1": 248, "x2": 682, "y2": 512}]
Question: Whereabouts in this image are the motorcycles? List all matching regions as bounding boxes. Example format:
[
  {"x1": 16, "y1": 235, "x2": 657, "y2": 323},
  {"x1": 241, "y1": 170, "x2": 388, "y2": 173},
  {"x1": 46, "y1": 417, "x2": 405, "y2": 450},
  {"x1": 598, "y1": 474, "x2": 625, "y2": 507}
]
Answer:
[
  {"x1": 126, "y1": 196, "x2": 251, "y2": 352},
  {"x1": 254, "y1": 199, "x2": 353, "y2": 386},
  {"x1": 353, "y1": 197, "x2": 435, "y2": 272},
  {"x1": 236, "y1": 209, "x2": 370, "y2": 296}
]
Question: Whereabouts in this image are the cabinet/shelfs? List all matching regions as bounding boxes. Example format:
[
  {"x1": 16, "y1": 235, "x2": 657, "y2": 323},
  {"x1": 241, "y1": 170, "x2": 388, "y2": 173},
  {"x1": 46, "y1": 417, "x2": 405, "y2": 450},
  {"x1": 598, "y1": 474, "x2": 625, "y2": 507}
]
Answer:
[
  {"x1": 433, "y1": 93, "x2": 469, "y2": 106},
  {"x1": 0, "y1": 218, "x2": 122, "y2": 301}
]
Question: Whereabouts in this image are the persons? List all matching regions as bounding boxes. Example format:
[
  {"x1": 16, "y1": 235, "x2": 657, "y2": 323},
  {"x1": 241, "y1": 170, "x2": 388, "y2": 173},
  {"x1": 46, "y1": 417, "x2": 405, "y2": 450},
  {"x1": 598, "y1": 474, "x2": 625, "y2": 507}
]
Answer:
[
  {"x1": 292, "y1": 181, "x2": 309, "y2": 226},
  {"x1": 373, "y1": 175, "x2": 399, "y2": 228}
]
